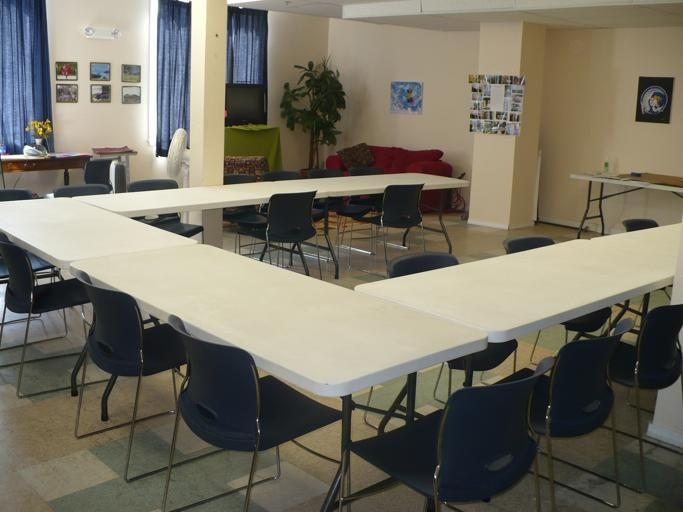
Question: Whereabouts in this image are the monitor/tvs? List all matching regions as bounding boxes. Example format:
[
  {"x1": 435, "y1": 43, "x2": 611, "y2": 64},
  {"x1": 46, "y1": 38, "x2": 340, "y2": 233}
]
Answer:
[{"x1": 225, "y1": 83, "x2": 268, "y2": 126}]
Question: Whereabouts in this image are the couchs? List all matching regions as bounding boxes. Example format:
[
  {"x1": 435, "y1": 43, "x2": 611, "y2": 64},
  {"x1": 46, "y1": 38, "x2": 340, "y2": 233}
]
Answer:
[{"x1": 326, "y1": 146, "x2": 452, "y2": 213}]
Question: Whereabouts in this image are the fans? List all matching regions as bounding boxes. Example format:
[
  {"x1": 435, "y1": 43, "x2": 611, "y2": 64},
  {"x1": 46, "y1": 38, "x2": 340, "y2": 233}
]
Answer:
[{"x1": 167, "y1": 129, "x2": 190, "y2": 224}]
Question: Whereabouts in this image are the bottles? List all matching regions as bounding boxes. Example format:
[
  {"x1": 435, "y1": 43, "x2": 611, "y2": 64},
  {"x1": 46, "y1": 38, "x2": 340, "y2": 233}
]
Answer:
[{"x1": 603, "y1": 161, "x2": 608, "y2": 176}]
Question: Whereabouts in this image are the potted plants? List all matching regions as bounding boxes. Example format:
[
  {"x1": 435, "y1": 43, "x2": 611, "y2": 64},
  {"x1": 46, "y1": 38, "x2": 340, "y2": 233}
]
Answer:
[{"x1": 279, "y1": 49, "x2": 349, "y2": 179}]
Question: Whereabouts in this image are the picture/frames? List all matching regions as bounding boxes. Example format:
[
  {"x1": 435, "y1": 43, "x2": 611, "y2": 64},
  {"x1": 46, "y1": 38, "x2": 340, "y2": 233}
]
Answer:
[
  {"x1": 122, "y1": 64, "x2": 141, "y2": 83},
  {"x1": 90, "y1": 62, "x2": 111, "y2": 81},
  {"x1": 122, "y1": 86, "x2": 141, "y2": 104},
  {"x1": 56, "y1": 84, "x2": 78, "y2": 103},
  {"x1": 55, "y1": 62, "x2": 78, "y2": 80},
  {"x1": 90, "y1": 84, "x2": 111, "y2": 103}
]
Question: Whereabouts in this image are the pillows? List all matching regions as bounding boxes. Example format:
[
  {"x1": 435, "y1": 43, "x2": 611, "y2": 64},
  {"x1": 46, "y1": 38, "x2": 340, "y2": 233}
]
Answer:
[{"x1": 336, "y1": 143, "x2": 374, "y2": 174}]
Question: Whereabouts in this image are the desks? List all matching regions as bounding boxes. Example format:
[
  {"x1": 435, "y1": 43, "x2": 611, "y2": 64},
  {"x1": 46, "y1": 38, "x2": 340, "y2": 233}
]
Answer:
[{"x1": 2, "y1": 151, "x2": 93, "y2": 185}]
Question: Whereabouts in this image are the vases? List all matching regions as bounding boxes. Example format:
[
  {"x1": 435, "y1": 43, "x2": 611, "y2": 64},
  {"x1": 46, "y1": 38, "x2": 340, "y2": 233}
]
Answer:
[{"x1": 35, "y1": 138, "x2": 43, "y2": 145}]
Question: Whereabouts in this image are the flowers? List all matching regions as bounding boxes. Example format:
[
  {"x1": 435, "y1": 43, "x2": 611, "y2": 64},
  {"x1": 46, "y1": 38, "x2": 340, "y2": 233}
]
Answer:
[{"x1": 25, "y1": 118, "x2": 52, "y2": 138}]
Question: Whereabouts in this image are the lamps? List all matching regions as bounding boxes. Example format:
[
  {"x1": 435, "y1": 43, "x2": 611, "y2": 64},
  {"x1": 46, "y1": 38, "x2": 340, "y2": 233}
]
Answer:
[{"x1": 79, "y1": 22, "x2": 123, "y2": 40}]
